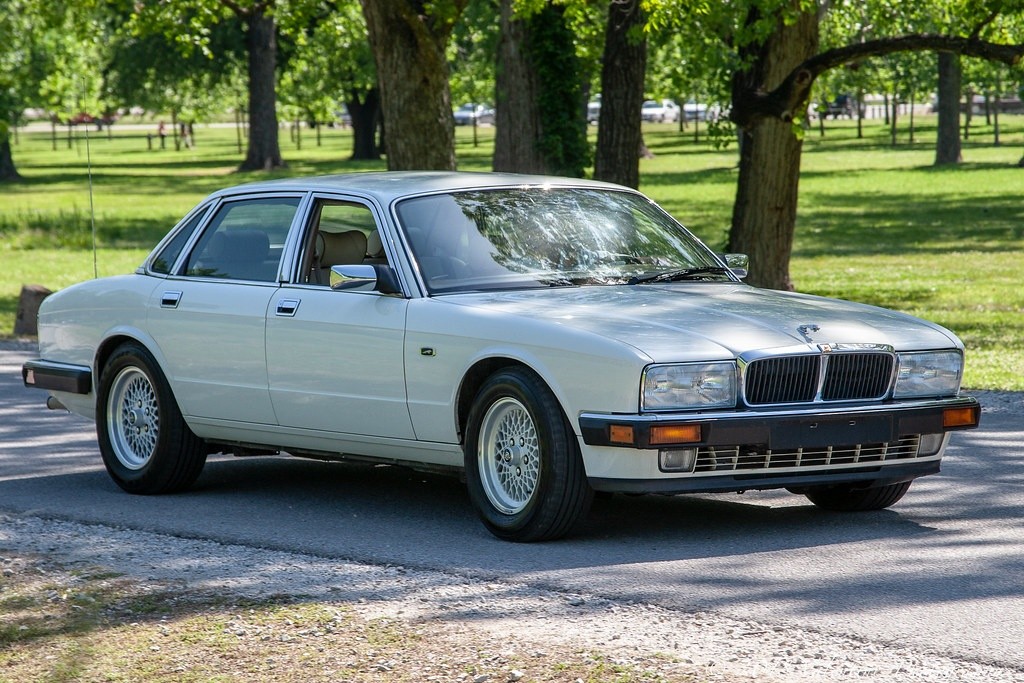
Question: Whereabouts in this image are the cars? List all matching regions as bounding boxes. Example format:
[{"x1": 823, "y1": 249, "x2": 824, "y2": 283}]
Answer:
[
  {"x1": 22, "y1": 171, "x2": 982, "y2": 542},
  {"x1": 642, "y1": 98, "x2": 732, "y2": 124},
  {"x1": 820, "y1": 96, "x2": 866, "y2": 119},
  {"x1": 454, "y1": 103, "x2": 496, "y2": 124}
]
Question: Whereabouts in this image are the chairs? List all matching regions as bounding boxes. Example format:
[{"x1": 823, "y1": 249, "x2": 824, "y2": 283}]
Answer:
[{"x1": 310, "y1": 230, "x2": 368, "y2": 285}]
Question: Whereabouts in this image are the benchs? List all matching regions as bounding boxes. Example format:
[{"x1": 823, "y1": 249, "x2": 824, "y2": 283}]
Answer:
[{"x1": 196, "y1": 227, "x2": 467, "y2": 282}]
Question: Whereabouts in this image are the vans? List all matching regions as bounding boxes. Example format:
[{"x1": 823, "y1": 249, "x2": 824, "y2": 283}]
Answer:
[{"x1": 586, "y1": 93, "x2": 602, "y2": 123}]
